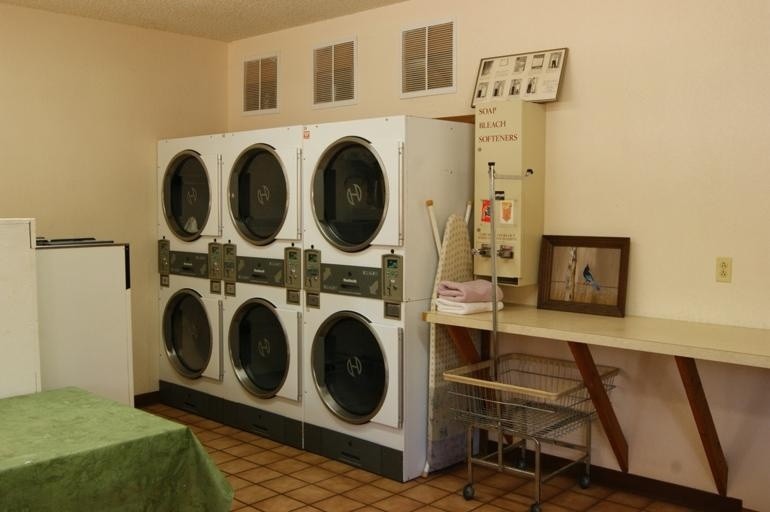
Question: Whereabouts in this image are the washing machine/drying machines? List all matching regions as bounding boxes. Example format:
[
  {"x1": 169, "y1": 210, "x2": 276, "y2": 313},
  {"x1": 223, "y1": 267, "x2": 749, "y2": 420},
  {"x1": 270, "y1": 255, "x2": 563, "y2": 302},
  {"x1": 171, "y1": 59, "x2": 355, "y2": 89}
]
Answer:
[
  {"x1": 227, "y1": 271, "x2": 304, "y2": 450},
  {"x1": 302, "y1": 112, "x2": 471, "y2": 298},
  {"x1": 218, "y1": 126, "x2": 299, "y2": 269},
  {"x1": 151, "y1": 137, "x2": 224, "y2": 262},
  {"x1": 302, "y1": 274, "x2": 419, "y2": 484},
  {"x1": 154, "y1": 266, "x2": 225, "y2": 418}
]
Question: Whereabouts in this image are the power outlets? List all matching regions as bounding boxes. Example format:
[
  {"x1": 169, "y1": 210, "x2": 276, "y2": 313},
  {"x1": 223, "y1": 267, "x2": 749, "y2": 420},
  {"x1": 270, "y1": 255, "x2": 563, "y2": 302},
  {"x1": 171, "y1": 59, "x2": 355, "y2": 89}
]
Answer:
[{"x1": 715, "y1": 257, "x2": 732, "y2": 283}]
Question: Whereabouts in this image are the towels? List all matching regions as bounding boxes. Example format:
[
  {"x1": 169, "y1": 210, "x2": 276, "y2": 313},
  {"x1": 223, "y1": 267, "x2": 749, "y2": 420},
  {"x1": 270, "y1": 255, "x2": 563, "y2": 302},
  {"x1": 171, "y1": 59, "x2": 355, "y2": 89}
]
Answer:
[
  {"x1": 433, "y1": 300, "x2": 505, "y2": 314},
  {"x1": 437, "y1": 279, "x2": 504, "y2": 315},
  {"x1": 435, "y1": 280, "x2": 503, "y2": 302}
]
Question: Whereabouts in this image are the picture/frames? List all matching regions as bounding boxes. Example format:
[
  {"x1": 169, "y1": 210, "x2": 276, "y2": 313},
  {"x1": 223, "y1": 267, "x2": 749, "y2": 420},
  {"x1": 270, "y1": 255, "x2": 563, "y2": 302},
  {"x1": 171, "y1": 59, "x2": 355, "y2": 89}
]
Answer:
[
  {"x1": 536, "y1": 234, "x2": 630, "y2": 318},
  {"x1": 471, "y1": 48, "x2": 568, "y2": 104}
]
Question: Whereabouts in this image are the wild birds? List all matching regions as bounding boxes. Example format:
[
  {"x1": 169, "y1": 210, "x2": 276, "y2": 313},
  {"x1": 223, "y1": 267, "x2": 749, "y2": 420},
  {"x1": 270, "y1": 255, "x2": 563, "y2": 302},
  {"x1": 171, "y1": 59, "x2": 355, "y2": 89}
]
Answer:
[{"x1": 583, "y1": 263, "x2": 594, "y2": 287}]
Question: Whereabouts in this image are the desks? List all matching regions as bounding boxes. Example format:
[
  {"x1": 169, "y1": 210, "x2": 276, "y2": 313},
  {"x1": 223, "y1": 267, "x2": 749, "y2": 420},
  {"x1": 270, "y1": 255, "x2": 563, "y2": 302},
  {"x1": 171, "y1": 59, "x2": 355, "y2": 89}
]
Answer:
[{"x1": 425, "y1": 303, "x2": 770, "y2": 495}]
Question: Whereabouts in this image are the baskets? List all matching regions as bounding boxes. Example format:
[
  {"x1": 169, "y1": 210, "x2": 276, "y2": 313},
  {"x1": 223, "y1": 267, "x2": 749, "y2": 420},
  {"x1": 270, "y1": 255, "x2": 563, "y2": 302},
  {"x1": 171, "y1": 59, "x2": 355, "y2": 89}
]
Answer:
[{"x1": 443, "y1": 352, "x2": 620, "y2": 442}]
